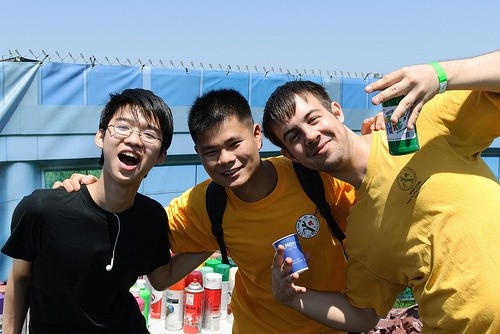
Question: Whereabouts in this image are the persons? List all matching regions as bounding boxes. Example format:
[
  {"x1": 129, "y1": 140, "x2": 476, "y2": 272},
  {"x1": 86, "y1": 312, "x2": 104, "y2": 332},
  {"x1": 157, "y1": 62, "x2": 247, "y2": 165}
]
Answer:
[
  {"x1": 261, "y1": 50, "x2": 500, "y2": 334},
  {"x1": 0, "y1": 88, "x2": 216, "y2": 334},
  {"x1": 51, "y1": 88, "x2": 388, "y2": 334}
]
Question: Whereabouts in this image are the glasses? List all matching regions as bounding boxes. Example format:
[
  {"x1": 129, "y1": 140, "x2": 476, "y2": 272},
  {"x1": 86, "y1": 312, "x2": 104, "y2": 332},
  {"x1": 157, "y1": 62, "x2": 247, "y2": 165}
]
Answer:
[{"x1": 102, "y1": 123, "x2": 162, "y2": 144}]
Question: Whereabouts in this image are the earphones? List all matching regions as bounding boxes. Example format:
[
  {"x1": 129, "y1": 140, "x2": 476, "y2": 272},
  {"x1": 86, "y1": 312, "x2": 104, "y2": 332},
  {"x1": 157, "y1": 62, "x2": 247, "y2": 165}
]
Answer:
[{"x1": 106, "y1": 258, "x2": 114, "y2": 271}]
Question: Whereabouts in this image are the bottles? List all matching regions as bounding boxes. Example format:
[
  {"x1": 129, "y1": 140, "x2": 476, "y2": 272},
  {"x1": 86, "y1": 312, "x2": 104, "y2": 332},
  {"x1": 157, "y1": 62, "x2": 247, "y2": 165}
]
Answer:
[
  {"x1": 130, "y1": 288, "x2": 144, "y2": 314},
  {"x1": 131, "y1": 279, "x2": 150, "y2": 331},
  {"x1": 183, "y1": 278, "x2": 205, "y2": 334},
  {"x1": 383, "y1": 82, "x2": 420, "y2": 156}
]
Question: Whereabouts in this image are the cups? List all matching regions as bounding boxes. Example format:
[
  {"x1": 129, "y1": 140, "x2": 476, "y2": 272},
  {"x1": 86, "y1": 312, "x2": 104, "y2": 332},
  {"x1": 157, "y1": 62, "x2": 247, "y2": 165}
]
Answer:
[{"x1": 271, "y1": 233, "x2": 309, "y2": 276}]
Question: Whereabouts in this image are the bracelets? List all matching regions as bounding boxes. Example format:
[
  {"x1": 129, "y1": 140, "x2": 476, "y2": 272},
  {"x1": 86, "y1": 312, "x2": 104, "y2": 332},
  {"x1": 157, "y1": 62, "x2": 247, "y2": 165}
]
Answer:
[{"x1": 430, "y1": 61, "x2": 447, "y2": 94}]
